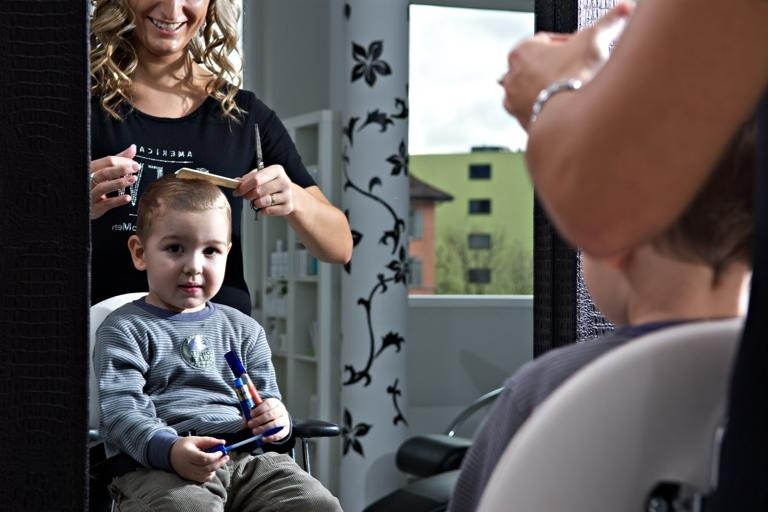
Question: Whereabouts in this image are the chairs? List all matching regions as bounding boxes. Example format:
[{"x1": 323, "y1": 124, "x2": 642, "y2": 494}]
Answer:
[{"x1": 476, "y1": 319, "x2": 745, "y2": 512}]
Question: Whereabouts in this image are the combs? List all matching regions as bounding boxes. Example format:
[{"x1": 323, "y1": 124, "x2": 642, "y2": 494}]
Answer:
[{"x1": 177, "y1": 168, "x2": 241, "y2": 188}]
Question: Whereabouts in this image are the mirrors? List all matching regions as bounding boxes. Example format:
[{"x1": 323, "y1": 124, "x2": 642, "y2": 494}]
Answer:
[{"x1": 2, "y1": 0, "x2": 578, "y2": 511}]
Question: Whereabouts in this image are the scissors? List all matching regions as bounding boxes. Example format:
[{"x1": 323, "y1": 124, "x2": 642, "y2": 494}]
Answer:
[{"x1": 251, "y1": 124, "x2": 263, "y2": 211}]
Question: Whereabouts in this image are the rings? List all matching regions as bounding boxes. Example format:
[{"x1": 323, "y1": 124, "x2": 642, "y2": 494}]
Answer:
[
  {"x1": 271, "y1": 194, "x2": 275, "y2": 205},
  {"x1": 92, "y1": 172, "x2": 98, "y2": 186}
]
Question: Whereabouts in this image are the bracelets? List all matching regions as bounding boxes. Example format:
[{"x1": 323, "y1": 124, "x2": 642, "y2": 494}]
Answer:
[{"x1": 532, "y1": 80, "x2": 580, "y2": 121}]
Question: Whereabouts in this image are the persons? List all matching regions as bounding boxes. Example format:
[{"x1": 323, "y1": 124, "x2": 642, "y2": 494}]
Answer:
[
  {"x1": 92, "y1": 180, "x2": 342, "y2": 511},
  {"x1": 497, "y1": 0, "x2": 766, "y2": 511},
  {"x1": 89, "y1": 1, "x2": 353, "y2": 312},
  {"x1": 448, "y1": 160, "x2": 745, "y2": 511}
]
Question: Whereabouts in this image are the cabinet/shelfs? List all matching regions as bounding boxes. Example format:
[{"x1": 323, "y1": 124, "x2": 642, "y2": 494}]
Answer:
[{"x1": 240, "y1": 108, "x2": 344, "y2": 495}]
